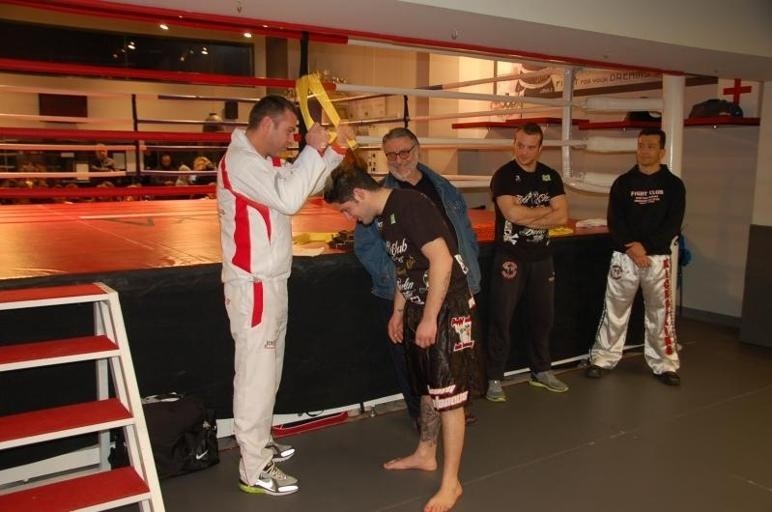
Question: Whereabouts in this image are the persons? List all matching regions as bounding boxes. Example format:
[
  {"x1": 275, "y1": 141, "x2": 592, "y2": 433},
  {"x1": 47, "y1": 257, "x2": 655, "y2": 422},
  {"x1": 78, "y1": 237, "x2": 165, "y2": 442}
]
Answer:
[
  {"x1": 0, "y1": 144, "x2": 217, "y2": 206},
  {"x1": 352, "y1": 128, "x2": 483, "y2": 435},
  {"x1": 217, "y1": 95, "x2": 354, "y2": 497},
  {"x1": 483, "y1": 121, "x2": 568, "y2": 402},
  {"x1": 323, "y1": 163, "x2": 475, "y2": 511},
  {"x1": 587, "y1": 129, "x2": 685, "y2": 385}
]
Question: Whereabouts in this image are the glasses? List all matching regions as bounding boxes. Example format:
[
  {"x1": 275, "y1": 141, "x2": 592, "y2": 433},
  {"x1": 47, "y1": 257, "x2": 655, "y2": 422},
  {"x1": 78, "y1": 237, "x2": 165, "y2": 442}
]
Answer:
[{"x1": 385, "y1": 146, "x2": 415, "y2": 161}]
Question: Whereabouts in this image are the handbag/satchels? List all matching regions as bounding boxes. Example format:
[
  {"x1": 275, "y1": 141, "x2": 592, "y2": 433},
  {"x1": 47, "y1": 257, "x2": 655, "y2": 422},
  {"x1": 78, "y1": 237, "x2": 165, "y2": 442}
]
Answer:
[{"x1": 108, "y1": 392, "x2": 220, "y2": 480}]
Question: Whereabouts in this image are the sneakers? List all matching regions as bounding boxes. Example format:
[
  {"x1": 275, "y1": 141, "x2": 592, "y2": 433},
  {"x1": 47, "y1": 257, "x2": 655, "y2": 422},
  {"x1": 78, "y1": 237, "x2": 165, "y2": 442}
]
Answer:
[
  {"x1": 238, "y1": 461, "x2": 299, "y2": 496},
  {"x1": 529, "y1": 370, "x2": 569, "y2": 393},
  {"x1": 485, "y1": 379, "x2": 507, "y2": 403},
  {"x1": 585, "y1": 365, "x2": 605, "y2": 378},
  {"x1": 660, "y1": 372, "x2": 680, "y2": 385},
  {"x1": 265, "y1": 435, "x2": 295, "y2": 462}
]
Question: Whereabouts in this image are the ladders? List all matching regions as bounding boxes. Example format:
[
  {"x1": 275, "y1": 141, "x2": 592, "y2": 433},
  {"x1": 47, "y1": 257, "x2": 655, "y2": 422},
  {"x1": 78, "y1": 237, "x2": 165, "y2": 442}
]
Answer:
[{"x1": 0, "y1": 281, "x2": 166, "y2": 512}]
